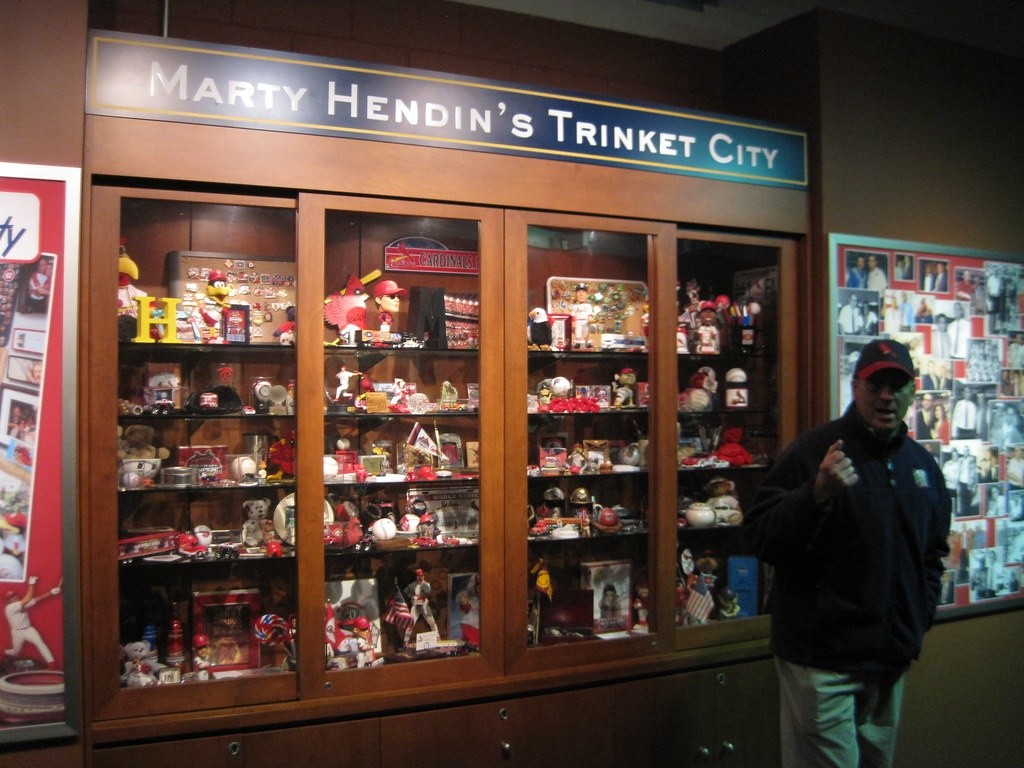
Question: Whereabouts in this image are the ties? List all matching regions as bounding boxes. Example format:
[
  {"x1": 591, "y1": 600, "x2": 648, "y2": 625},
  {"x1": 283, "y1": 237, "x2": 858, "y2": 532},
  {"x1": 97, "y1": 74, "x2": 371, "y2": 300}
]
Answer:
[
  {"x1": 990, "y1": 568, "x2": 993, "y2": 589},
  {"x1": 965, "y1": 407, "x2": 968, "y2": 430},
  {"x1": 851, "y1": 308, "x2": 854, "y2": 333},
  {"x1": 954, "y1": 323, "x2": 959, "y2": 354}
]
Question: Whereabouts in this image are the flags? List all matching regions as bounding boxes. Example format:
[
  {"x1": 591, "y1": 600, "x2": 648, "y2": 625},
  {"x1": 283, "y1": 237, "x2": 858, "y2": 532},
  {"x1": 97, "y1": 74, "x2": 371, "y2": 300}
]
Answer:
[
  {"x1": 326, "y1": 604, "x2": 346, "y2": 650},
  {"x1": 686, "y1": 574, "x2": 714, "y2": 624},
  {"x1": 383, "y1": 584, "x2": 414, "y2": 631}
]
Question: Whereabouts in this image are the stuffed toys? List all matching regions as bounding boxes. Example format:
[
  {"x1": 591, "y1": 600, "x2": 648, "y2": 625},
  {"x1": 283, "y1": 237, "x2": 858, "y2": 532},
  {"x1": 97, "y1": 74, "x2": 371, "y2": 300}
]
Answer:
[
  {"x1": 528, "y1": 308, "x2": 552, "y2": 350},
  {"x1": 239, "y1": 497, "x2": 272, "y2": 546},
  {"x1": 704, "y1": 476, "x2": 743, "y2": 525},
  {"x1": 121, "y1": 640, "x2": 167, "y2": 687},
  {"x1": 117, "y1": 423, "x2": 170, "y2": 459},
  {"x1": 272, "y1": 305, "x2": 297, "y2": 345}
]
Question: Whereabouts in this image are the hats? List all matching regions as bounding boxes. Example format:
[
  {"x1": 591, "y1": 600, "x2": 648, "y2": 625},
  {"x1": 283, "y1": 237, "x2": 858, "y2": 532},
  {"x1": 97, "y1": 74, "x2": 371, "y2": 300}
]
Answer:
[{"x1": 853, "y1": 338, "x2": 914, "y2": 382}]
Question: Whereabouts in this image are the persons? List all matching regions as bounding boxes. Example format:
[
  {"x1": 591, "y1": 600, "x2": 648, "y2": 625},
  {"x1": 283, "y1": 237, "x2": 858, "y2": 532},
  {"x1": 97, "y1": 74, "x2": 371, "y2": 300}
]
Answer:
[
  {"x1": 570, "y1": 283, "x2": 593, "y2": 349},
  {"x1": 568, "y1": 444, "x2": 585, "y2": 468},
  {"x1": 333, "y1": 365, "x2": 358, "y2": 401},
  {"x1": 697, "y1": 301, "x2": 719, "y2": 346},
  {"x1": 28, "y1": 258, "x2": 53, "y2": 314},
  {"x1": 372, "y1": 280, "x2": 407, "y2": 332},
  {"x1": 353, "y1": 616, "x2": 374, "y2": 668},
  {"x1": 286, "y1": 383, "x2": 295, "y2": 415},
  {"x1": 736, "y1": 340, "x2": 952, "y2": 768},
  {"x1": 7, "y1": 406, "x2": 26, "y2": 441},
  {"x1": 0, "y1": 575, "x2": 62, "y2": 671},
  {"x1": 640, "y1": 294, "x2": 649, "y2": 349},
  {"x1": 32, "y1": 362, "x2": 41, "y2": 381},
  {"x1": 613, "y1": 368, "x2": 636, "y2": 406},
  {"x1": 838, "y1": 255, "x2": 1024, "y2": 605},
  {"x1": 405, "y1": 569, "x2": 439, "y2": 647},
  {"x1": 536, "y1": 379, "x2": 552, "y2": 405}
]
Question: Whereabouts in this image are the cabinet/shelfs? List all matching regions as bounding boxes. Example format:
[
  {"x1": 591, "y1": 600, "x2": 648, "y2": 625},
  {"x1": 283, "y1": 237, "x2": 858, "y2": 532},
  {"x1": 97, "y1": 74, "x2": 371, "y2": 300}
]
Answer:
[{"x1": 84, "y1": 172, "x2": 812, "y2": 768}]
